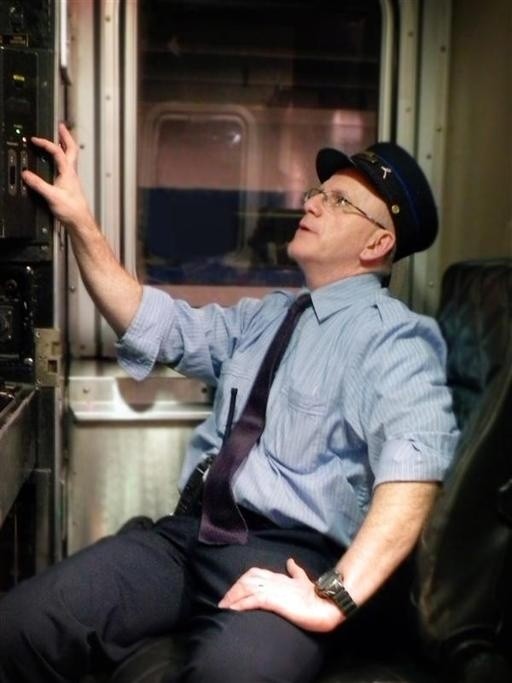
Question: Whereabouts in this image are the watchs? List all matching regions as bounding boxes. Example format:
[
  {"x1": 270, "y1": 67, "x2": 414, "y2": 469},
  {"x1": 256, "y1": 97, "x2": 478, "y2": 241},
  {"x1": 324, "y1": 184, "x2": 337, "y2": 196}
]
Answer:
[{"x1": 314, "y1": 568, "x2": 359, "y2": 618}]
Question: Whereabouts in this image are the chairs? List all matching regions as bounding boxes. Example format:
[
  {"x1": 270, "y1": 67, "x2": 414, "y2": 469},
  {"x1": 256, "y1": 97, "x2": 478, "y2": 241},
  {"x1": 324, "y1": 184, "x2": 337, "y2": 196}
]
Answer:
[{"x1": 113, "y1": 253, "x2": 510, "y2": 681}]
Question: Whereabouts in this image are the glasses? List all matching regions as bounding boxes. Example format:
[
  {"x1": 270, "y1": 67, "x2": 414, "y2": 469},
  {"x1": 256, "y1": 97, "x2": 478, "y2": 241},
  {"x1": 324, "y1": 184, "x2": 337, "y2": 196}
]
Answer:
[{"x1": 303, "y1": 188, "x2": 386, "y2": 231}]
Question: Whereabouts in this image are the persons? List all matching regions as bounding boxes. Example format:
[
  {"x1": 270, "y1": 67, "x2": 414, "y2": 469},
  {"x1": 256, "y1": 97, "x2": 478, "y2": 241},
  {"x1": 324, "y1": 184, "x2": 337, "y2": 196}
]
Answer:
[{"x1": 0, "y1": 122, "x2": 460, "y2": 683}]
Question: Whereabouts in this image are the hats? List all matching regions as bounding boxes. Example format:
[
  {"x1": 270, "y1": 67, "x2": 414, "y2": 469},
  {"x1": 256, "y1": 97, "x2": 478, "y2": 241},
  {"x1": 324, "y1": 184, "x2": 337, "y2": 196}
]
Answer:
[{"x1": 315, "y1": 142, "x2": 438, "y2": 261}]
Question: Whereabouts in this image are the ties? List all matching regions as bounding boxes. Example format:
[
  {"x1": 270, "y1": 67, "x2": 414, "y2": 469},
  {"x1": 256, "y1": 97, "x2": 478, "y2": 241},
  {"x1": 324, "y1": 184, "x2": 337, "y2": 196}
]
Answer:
[{"x1": 200, "y1": 294, "x2": 311, "y2": 545}]
